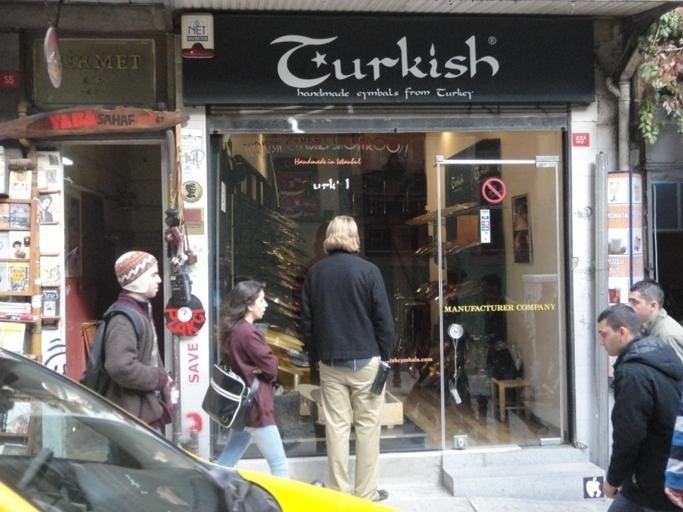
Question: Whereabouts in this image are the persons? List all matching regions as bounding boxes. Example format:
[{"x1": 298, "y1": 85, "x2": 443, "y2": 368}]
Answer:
[
  {"x1": 100, "y1": 251, "x2": 178, "y2": 434},
  {"x1": 298, "y1": 213, "x2": 390, "y2": 504},
  {"x1": 595, "y1": 306, "x2": 682, "y2": 511},
  {"x1": 623, "y1": 282, "x2": 682, "y2": 362},
  {"x1": 211, "y1": 282, "x2": 289, "y2": 478}
]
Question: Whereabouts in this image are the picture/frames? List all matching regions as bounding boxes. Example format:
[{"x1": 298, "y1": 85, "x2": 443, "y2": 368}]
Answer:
[{"x1": 512, "y1": 193, "x2": 532, "y2": 264}]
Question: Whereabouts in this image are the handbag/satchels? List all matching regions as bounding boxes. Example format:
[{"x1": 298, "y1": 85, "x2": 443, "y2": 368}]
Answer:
[{"x1": 370, "y1": 362, "x2": 392, "y2": 395}]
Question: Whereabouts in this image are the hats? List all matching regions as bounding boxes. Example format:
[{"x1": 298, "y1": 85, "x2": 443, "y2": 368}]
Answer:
[{"x1": 114, "y1": 250, "x2": 158, "y2": 287}]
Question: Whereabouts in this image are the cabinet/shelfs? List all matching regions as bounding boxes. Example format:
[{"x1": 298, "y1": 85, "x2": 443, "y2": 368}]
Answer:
[{"x1": 351, "y1": 171, "x2": 429, "y2": 256}]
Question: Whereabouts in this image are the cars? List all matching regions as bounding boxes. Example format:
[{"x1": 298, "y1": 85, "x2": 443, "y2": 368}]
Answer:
[{"x1": 1, "y1": 348, "x2": 386, "y2": 507}]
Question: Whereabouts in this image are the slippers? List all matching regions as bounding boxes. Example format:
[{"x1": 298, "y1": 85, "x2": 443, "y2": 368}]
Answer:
[{"x1": 373, "y1": 490, "x2": 389, "y2": 503}]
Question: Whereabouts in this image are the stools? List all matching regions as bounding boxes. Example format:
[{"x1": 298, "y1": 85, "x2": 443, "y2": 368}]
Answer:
[{"x1": 490, "y1": 377, "x2": 531, "y2": 424}]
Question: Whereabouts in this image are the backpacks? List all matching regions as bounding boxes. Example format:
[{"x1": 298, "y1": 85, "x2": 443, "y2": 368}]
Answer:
[
  {"x1": 202, "y1": 363, "x2": 253, "y2": 433},
  {"x1": 79, "y1": 306, "x2": 144, "y2": 396}
]
